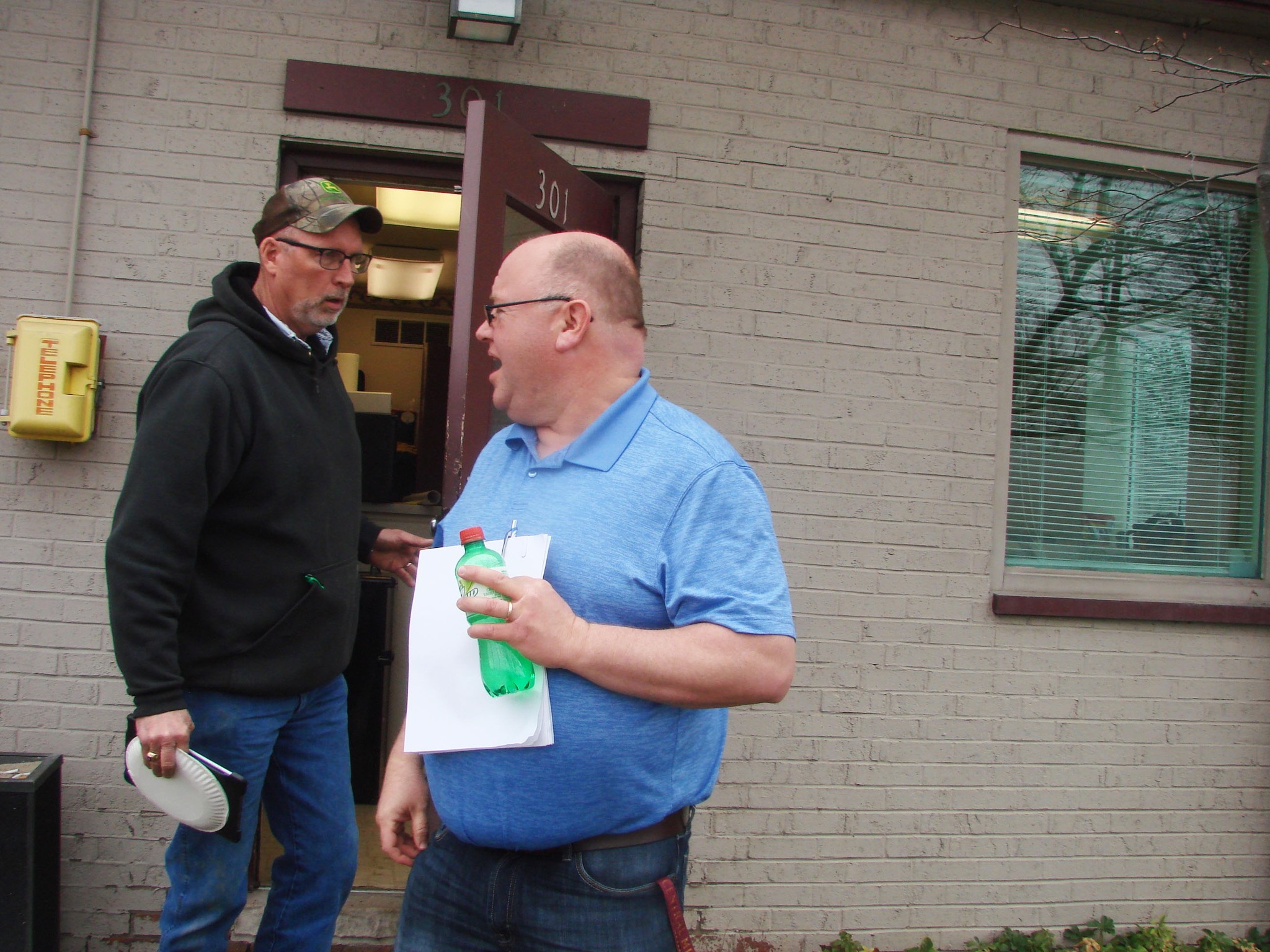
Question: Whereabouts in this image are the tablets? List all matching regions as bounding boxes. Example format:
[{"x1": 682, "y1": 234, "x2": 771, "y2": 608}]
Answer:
[{"x1": 124, "y1": 713, "x2": 248, "y2": 842}]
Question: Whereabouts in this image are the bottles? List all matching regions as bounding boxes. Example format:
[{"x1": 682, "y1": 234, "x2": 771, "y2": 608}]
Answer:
[{"x1": 456, "y1": 526, "x2": 536, "y2": 697}]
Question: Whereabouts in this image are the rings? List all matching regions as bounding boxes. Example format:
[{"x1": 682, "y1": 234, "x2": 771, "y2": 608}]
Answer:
[
  {"x1": 147, "y1": 752, "x2": 161, "y2": 761},
  {"x1": 405, "y1": 563, "x2": 412, "y2": 570},
  {"x1": 505, "y1": 600, "x2": 513, "y2": 621}
]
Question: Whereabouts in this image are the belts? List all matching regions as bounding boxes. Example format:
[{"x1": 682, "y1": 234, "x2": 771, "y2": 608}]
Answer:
[{"x1": 574, "y1": 805, "x2": 690, "y2": 854}]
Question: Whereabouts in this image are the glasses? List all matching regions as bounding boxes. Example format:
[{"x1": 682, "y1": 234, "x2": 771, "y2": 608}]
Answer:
[
  {"x1": 483, "y1": 296, "x2": 594, "y2": 326},
  {"x1": 275, "y1": 238, "x2": 372, "y2": 274}
]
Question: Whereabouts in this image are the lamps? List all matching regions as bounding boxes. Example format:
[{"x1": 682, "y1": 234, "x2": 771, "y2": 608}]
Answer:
[
  {"x1": 446, "y1": 0, "x2": 522, "y2": 46},
  {"x1": 376, "y1": 186, "x2": 461, "y2": 231},
  {"x1": 366, "y1": 246, "x2": 447, "y2": 301}
]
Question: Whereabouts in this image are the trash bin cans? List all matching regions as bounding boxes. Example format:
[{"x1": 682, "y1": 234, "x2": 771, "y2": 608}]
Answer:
[{"x1": 0, "y1": 751, "x2": 65, "y2": 952}]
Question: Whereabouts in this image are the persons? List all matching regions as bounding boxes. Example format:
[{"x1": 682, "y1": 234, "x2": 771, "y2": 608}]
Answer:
[
  {"x1": 106, "y1": 179, "x2": 432, "y2": 952},
  {"x1": 375, "y1": 232, "x2": 793, "y2": 952}
]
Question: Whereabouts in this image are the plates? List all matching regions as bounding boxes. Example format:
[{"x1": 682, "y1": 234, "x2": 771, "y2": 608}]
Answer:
[{"x1": 125, "y1": 736, "x2": 228, "y2": 832}]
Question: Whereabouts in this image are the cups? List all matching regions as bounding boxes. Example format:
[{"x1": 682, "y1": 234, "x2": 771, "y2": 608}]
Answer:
[{"x1": 336, "y1": 352, "x2": 360, "y2": 390}]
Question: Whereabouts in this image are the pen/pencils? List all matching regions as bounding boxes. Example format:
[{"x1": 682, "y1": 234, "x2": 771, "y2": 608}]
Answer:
[
  {"x1": 501, "y1": 519, "x2": 517, "y2": 557},
  {"x1": 188, "y1": 749, "x2": 245, "y2": 783}
]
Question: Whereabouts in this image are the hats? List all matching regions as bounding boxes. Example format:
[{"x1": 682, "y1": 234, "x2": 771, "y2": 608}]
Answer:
[{"x1": 252, "y1": 177, "x2": 382, "y2": 248}]
